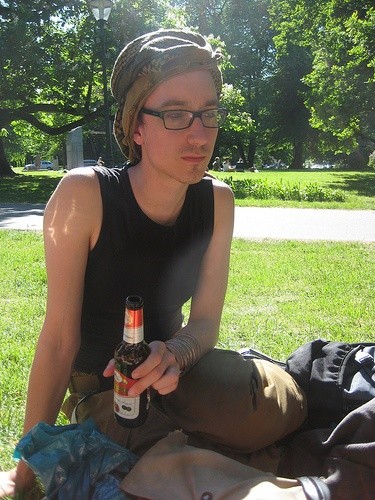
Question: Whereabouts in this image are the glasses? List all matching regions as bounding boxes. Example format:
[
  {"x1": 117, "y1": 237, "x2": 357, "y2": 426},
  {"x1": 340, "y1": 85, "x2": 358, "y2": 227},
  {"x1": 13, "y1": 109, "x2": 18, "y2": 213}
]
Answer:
[{"x1": 140, "y1": 106, "x2": 230, "y2": 131}]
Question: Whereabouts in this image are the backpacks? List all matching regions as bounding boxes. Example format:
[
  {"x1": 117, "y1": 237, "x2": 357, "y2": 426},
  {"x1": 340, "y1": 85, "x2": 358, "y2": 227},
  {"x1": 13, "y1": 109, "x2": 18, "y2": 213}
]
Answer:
[{"x1": 287, "y1": 338, "x2": 375, "y2": 427}]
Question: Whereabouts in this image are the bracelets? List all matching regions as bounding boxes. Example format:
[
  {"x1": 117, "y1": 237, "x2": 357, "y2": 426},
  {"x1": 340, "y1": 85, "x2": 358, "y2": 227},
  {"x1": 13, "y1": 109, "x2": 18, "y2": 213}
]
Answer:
[{"x1": 163, "y1": 330, "x2": 205, "y2": 378}]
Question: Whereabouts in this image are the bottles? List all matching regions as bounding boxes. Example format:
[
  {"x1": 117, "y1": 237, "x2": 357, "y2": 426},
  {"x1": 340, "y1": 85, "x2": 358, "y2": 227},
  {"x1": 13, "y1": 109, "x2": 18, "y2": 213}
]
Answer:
[{"x1": 113, "y1": 295, "x2": 151, "y2": 428}]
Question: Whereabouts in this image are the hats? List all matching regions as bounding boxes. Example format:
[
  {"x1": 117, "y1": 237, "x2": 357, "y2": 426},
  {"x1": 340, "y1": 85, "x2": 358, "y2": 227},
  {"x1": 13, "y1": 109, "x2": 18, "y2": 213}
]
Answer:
[{"x1": 111, "y1": 29, "x2": 223, "y2": 160}]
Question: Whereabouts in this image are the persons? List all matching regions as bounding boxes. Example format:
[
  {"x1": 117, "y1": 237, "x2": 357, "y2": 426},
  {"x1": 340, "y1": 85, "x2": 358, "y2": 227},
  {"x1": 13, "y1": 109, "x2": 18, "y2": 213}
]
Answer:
[{"x1": 0, "y1": 30, "x2": 375, "y2": 499}]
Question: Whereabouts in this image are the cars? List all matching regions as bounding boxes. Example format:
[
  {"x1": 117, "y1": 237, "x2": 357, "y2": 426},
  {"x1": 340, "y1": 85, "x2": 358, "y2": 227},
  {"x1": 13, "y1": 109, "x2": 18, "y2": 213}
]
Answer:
[
  {"x1": 84, "y1": 159, "x2": 98, "y2": 166},
  {"x1": 24, "y1": 160, "x2": 53, "y2": 170}
]
